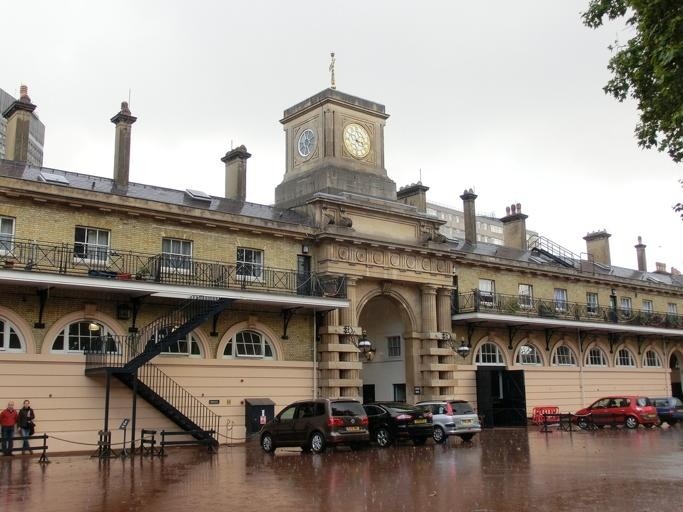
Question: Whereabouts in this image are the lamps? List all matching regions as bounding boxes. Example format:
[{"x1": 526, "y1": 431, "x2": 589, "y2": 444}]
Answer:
[
  {"x1": 440, "y1": 330, "x2": 469, "y2": 359},
  {"x1": 341, "y1": 325, "x2": 376, "y2": 363}
]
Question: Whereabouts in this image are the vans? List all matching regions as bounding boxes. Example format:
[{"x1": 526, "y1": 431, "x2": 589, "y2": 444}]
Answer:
[
  {"x1": 258, "y1": 397, "x2": 370, "y2": 455},
  {"x1": 574, "y1": 396, "x2": 660, "y2": 430},
  {"x1": 413, "y1": 400, "x2": 481, "y2": 444},
  {"x1": 647, "y1": 396, "x2": 682, "y2": 427}
]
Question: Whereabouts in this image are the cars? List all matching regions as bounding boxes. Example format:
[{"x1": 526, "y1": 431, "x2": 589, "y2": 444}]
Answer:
[{"x1": 361, "y1": 401, "x2": 434, "y2": 449}]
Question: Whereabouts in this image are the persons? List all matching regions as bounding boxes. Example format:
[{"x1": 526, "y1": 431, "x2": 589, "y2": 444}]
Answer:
[
  {"x1": 0, "y1": 401, "x2": 18, "y2": 456},
  {"x1": 17, "y1": 400, "x2": 35, "y2": 455}
]
